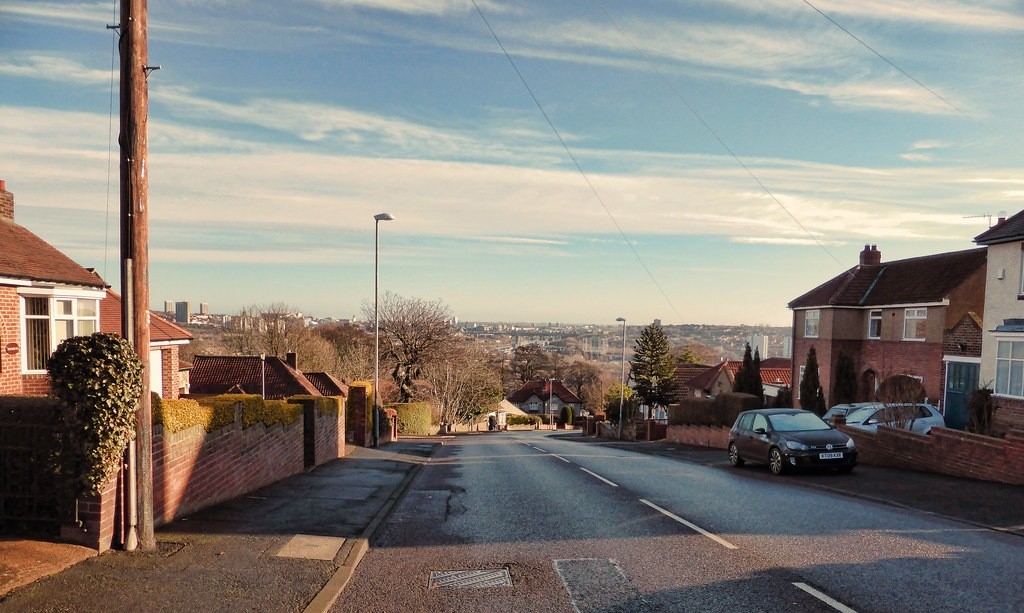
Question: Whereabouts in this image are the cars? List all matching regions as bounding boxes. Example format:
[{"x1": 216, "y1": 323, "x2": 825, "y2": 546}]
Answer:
[
  {"x1": 845, "y1": 403, "x2": 947, "y2": 436},
  {"x1": 822, "y1": 402, "x2": 883, "y2": 427},
  {"x1": 727, "y1": 409, "x2": 858, "y2": 475}
]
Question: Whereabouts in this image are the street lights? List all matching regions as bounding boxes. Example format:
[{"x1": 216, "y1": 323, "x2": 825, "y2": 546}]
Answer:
[
  {"x1": 371, "y1": 213, "x2": 395, "y2": 448},
  {"x1": 616, "y1": 317, "x2": 627, "y2": 438},
  {"x1": 549, "y1": 378, "x2": 555, "y2": 428}
]
